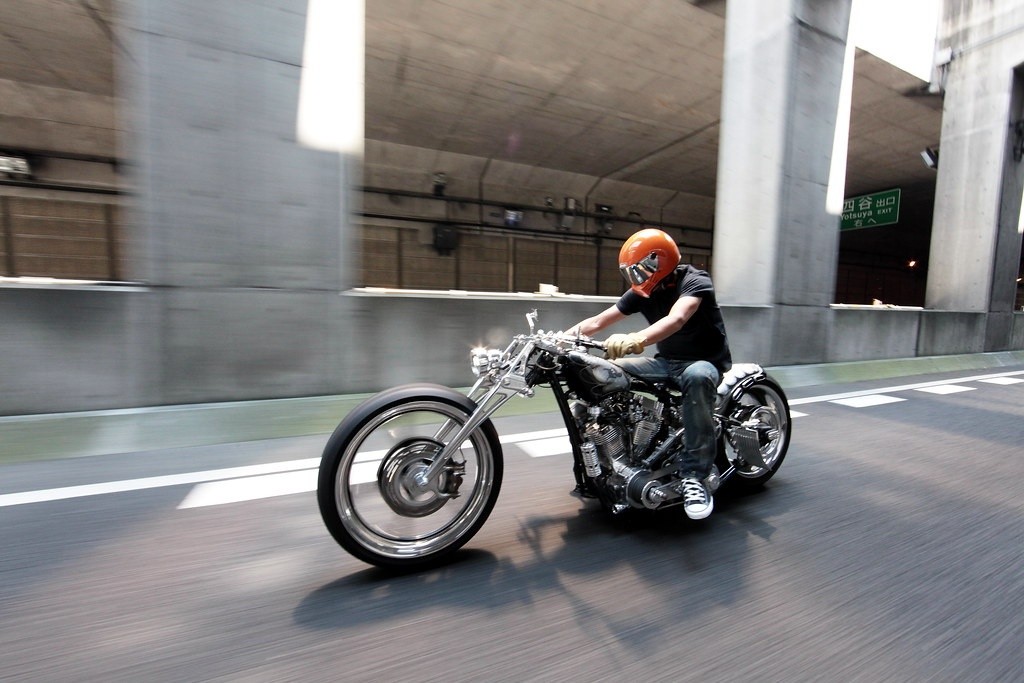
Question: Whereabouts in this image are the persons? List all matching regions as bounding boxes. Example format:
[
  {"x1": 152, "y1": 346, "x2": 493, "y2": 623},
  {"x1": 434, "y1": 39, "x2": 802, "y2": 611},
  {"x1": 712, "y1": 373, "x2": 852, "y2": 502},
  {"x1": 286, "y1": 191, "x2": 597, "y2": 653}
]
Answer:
[{"x1": 556, "y1": 228, "x2": 732, "y2": 520}]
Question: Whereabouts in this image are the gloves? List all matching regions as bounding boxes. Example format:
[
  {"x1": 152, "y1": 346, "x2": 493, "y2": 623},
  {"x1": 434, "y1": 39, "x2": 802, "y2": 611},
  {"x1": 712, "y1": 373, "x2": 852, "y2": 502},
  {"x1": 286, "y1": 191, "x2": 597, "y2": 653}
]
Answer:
[{"x1": 602, "y1": 332, "x2": 644, "y2": 360}]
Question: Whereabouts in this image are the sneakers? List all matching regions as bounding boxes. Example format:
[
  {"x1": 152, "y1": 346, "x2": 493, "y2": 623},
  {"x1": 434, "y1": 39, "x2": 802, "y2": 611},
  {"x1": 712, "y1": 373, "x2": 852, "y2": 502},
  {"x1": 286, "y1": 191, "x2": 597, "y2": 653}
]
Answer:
[{"x1": 681, "y1": 477, "x2": 713, "y2": 519}]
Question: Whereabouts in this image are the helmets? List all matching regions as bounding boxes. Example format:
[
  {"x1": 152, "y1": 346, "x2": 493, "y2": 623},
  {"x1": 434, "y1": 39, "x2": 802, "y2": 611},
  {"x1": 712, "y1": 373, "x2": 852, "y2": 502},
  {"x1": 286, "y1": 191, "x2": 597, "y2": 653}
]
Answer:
[{"x1": 618, "y1": 228, "x2": 681, "y2": 298}]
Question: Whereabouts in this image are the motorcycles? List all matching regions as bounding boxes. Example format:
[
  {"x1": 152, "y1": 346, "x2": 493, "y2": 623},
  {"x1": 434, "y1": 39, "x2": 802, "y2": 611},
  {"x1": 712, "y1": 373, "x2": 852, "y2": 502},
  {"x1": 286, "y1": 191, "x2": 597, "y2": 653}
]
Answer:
[{"x1": 317, "y1": 304, "x2": 792, "y2": 576}]
